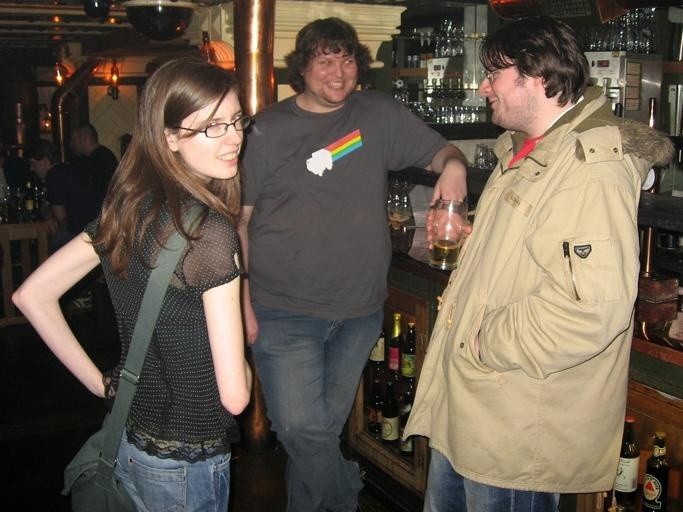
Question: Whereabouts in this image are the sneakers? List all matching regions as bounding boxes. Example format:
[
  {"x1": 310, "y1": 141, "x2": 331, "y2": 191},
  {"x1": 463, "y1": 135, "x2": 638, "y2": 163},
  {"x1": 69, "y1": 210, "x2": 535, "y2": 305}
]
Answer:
[{"x1": 65, "y1": 295, "x2": 92, "y2": 311}]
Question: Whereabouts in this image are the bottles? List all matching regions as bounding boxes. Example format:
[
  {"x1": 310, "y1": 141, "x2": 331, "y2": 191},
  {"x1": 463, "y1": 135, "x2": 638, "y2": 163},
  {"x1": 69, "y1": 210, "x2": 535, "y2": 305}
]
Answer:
[
  {"x1": 392, "y1": 89, "x2": 408, "y2": 107},
  {"x1": 419, "y1": 32, "x2": 426, "y2": 68},
  {"x1": 487, "y1": 148, "x2": 494, "y2": 168},
  {"x1": 431, "y1": 78, "x2": 437, "y2": 107},
  {"x1": 409, "y1": 102, "x2": 436, "y2": 122},
  {"x1": 448, "y1": 107, "x2": 454, "y2": 123},
  {"x1": 478, "y1": 147, "x2": 487, "y2": 169},
  {"x1": 425, "y1": 32, "x2": 433, "y2": 59},
  {"x1": 474, "y1": 143, "x2": 481, "y2": 167},
  {"x1": 401, "y1": 179, "x2": 409, "y2": 191},
  {"x1": 614, "y1": 103, "x2": 624, "y2": 117},
  {"x1": 642, "y1": 96, "x2": 660, "y2": 193},
  {"x1": 456, "y1": 77, "x2": 463, "y2": 105},
  {"x1": 381, "y1": 382, "x2": 399, "y2": 443},
  {"x1": 387, "y1": 313, "x2": 405, "y2": 373},
  {"x1": 387, "y1": 175, "x2": 392, "y2": 192},
  {"x1": 447, "y1": 77, "x2": 455, "y2": 106},
  {"x1": 464, "y1": 107, "x2": 468, "y2": 123},
  {"x1": 367, "y1": 378, "x2": 381, "y2": 434},
  {"x1": 369, "y1": 332, "x2": 385, "y2": 367},
  {"x1": 13, "y1": 187, "x2": 23, "y2": 222},
  {"x1": 33, "y1": 186, "x2": 43, "y2": 220},
  {"x1": 445, "y1": 107, "x2": 449, "y2": 124},
  {"x1": 441, "y1": 106, "x2": 445, "y2": 124},
  {"x1": 585, "y1": 7, "x2": 657, "y2": 54},
  {"x1": 454, "y1": 106, "x2": 458, "y2": 122},
  {"x1": 437, "y1": 108, "x2": 440, "y2": 122},
  {"x1": 392, "y1": 190, "x2": 400, "y2": 235},
  {"x1": 422, "y1": 78, "x2": 429, "y2": 102},
  {"x1": 435, "y1": 19, "x2": 463, "y2": 64},
  {"x1": 5, "y1": 186, "x2": 12, "y2": 210},
  {"x1": 470, "y1": 106, "x2": 475, "y2": 122},
  {"x1": 393, "y1": 178, "x2": 400, "y2": 195},
  {"x1": 387, "y1": 190, "x2": 392, "y2": 225},
  {"x1": 392, "y1": 27, "x2": 419, "y2": 68},
  {"x1": 614, "y1": 415, "x2": 641, "y2": 501},
  {"x1": 400, "y1": 322, "x2": 417, "y2": 379},
  {"x1": 20, "y1": 182, "x2": 38, "y2": 221},
  {"x1": 641, "y1": 431, "x2": 667, "y2": 512},
  {"x1": 437, "y1": 78, "x2": 447, "y2": 105},
  {"x1": 400, "y1": 187, "x2": 411, "y2": 234},
  {"x1": 475, "y1": 106, "x2": 479, "y2": 123},
  {"x1": 40, "y1": 191, "x2": 44, "y2": 203},
  {"x1": 2, "y1": 198, "x2": 11, "y2": 223},
  {"x1": 399, "y1": 391, "x2": 413, "y2": 454},
  {"x1": 459, "y1": 107, "x2": 464, "y2": 123}
]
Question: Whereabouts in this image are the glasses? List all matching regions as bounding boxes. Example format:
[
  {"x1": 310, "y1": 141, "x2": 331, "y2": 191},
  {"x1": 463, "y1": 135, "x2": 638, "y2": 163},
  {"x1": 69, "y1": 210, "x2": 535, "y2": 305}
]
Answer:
[
  {"x1": 484, "y1": 65, "x2": 516, "y2": 83},
  {"x1": 173, "y1": 115, "x2": 251, "y2": 138}
]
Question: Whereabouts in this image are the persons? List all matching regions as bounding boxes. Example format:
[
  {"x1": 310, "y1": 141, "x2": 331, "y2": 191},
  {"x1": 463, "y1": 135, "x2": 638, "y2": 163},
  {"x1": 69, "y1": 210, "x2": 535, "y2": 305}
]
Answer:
[
  {"x1": 402, "y1": 15, "x2": 676, "y2": 511},
  {"x1": 25, "y1": 140, "x2": 70, "y2": 256},
  {"x1": 236, "y1": 17, "x2": 468, "y2": 511},
  {"x1": 0, "y1": 142, "x2": 27, "y2": 202},
  {"x1": 69, "y1": 122, "x2": 119, "y2": 205},
  {"x1": 9, "y1": 54, "x2": 254, "y2": 512}
]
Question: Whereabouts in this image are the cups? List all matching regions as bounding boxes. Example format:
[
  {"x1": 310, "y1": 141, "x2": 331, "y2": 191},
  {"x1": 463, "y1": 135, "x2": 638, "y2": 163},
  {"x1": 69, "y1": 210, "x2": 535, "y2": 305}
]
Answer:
[{"x1": 428, "y1": 200, "x2": 468, "y2": 271}]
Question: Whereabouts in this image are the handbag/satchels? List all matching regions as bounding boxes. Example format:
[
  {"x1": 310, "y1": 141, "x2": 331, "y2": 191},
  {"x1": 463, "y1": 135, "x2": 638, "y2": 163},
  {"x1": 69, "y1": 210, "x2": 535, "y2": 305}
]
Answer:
[{"x1": 59, "y1": 428, "x2": 138, "y2": 512}]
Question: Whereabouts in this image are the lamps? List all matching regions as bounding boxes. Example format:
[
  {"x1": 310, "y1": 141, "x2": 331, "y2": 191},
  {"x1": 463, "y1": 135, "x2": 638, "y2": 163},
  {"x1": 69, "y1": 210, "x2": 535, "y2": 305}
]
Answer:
[
  {"x1": 108, "y1": 58, "x2": 120, "y2": 100},
  {"x1": 52, "y1": 60, "x2": 65, "y2": 88},
  {"x1": 38, "y1": 102, "x2": 52, "y2": 129}
]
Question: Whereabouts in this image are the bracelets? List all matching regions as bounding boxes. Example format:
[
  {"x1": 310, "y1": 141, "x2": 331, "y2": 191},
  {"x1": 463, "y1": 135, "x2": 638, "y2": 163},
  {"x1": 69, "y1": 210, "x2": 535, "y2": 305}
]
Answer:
[{"x1": 240, "y1": 272, "x2": 249, "y2": 279}]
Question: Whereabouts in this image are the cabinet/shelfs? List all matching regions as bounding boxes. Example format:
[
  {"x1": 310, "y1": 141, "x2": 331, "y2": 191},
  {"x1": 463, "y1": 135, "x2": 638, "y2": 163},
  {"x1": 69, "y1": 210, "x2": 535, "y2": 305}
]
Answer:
[{"x1": 342, "y1": 252, "x2": 683, "y2": 512}]
